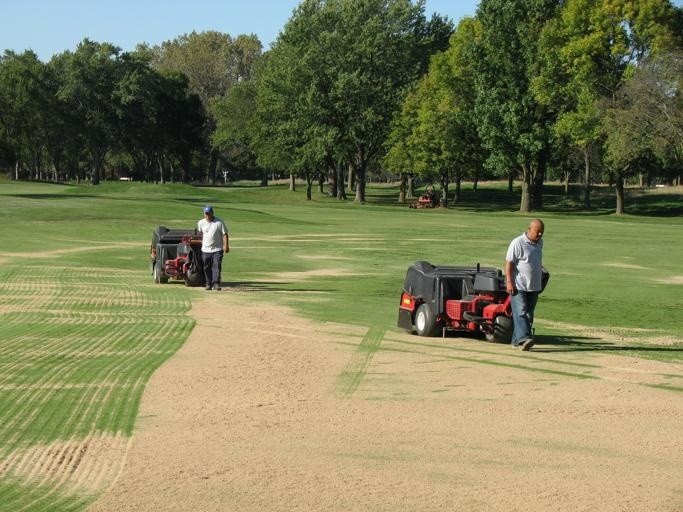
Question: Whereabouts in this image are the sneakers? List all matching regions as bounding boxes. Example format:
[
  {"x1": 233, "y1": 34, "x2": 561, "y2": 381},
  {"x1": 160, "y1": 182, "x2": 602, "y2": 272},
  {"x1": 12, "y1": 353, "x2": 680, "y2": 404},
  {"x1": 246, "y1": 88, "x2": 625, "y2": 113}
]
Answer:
[
  {"x1": 212, "y1": 282, "x2": 222, "y2": 290},
  {"x1": 521, "y1": 339, "x2": 535, "y2": 351},
  {"x1": 205, "y1": 283, "x2": 213, "y2": 290}
]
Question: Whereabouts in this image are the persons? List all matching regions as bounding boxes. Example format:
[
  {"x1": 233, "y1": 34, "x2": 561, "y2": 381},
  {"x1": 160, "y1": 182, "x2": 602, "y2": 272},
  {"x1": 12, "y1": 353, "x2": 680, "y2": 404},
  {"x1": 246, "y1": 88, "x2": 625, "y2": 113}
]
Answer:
[
  {"x1": 504, "y1": 218, "x2": 544, "y2": 350},
  {"x1": 196, "y1": 205, "x2": 229, "y2": 291}
]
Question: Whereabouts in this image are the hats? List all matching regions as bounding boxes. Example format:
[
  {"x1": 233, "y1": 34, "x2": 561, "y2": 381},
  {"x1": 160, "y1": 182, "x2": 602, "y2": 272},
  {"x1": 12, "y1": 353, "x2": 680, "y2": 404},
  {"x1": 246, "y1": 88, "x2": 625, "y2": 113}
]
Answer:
[{"x1": 204, "y1": 205, "x2": 213, "y2": 215}]
